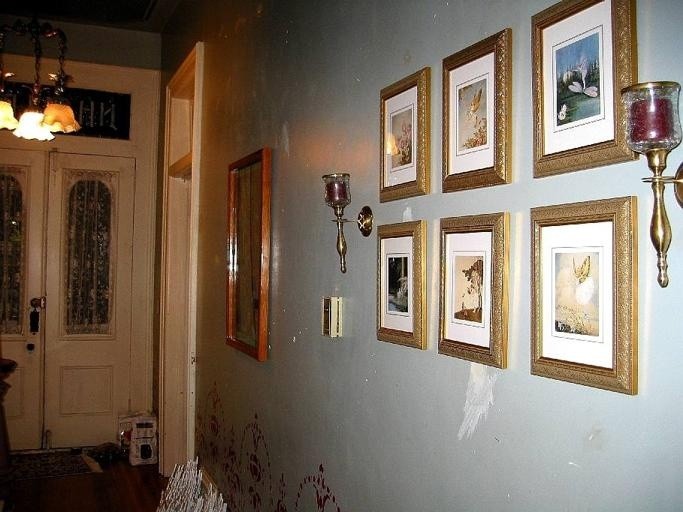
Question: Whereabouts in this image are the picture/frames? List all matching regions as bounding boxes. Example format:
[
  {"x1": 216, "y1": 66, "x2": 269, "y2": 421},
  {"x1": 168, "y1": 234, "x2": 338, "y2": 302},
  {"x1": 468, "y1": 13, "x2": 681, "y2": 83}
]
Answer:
[
  {"x1": 374, "y1": 218, "x2": 429, "y2": 353},
  {"x1": 440, "y1": 26, "x2": 513, "y2": 194},
  {"x1": 435, "y1": 210, "x2": 510, "y2": 372},
  {"x1": 528, "y1": 0, "x2": 643, "y2": 181},
  {"x1": 377, "y1": 65, "x2": 430, "y2": 203},
  {"x1": 528, "y1": 195, "x2": 640, "y2": 397}
]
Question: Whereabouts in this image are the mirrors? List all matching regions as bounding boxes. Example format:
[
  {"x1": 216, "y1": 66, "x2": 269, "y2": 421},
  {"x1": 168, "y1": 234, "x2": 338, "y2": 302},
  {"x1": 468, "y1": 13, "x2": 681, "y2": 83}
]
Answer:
[{"x1": 221, "y1": 145, "x2": 270, "y2": 364}]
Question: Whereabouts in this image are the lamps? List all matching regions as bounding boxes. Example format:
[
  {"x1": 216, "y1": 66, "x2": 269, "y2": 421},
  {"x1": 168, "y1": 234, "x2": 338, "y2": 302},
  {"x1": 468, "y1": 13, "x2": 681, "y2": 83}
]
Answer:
[
  {"x1": 617, "y1": 79, "x2": 682, "y2": 287},
  {"x1": 321, "y1": 172, "x2": 373, "y2": 274},
  {"x1": 0, "y1": 0, "x2": 81, "y2": 144}
]
